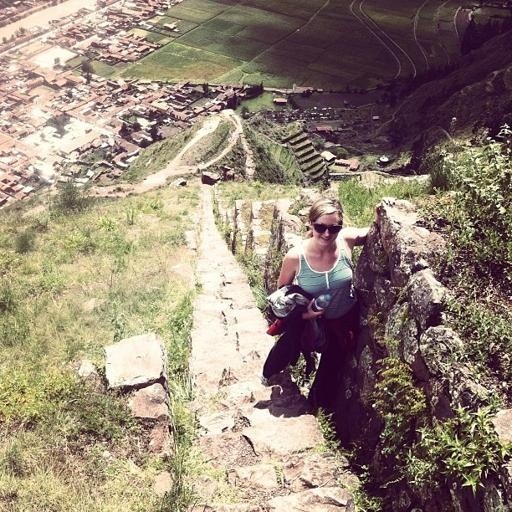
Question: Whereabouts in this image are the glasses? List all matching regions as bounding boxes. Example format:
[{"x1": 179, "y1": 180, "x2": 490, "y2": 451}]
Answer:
[{"x1": 313, "y1": 223, "x2": 342, "y2": 233}]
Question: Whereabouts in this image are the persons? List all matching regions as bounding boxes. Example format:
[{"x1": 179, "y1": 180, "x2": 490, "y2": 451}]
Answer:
[{"x1": 262, "y1": 196, "x2": 376, "y2": 380}]
